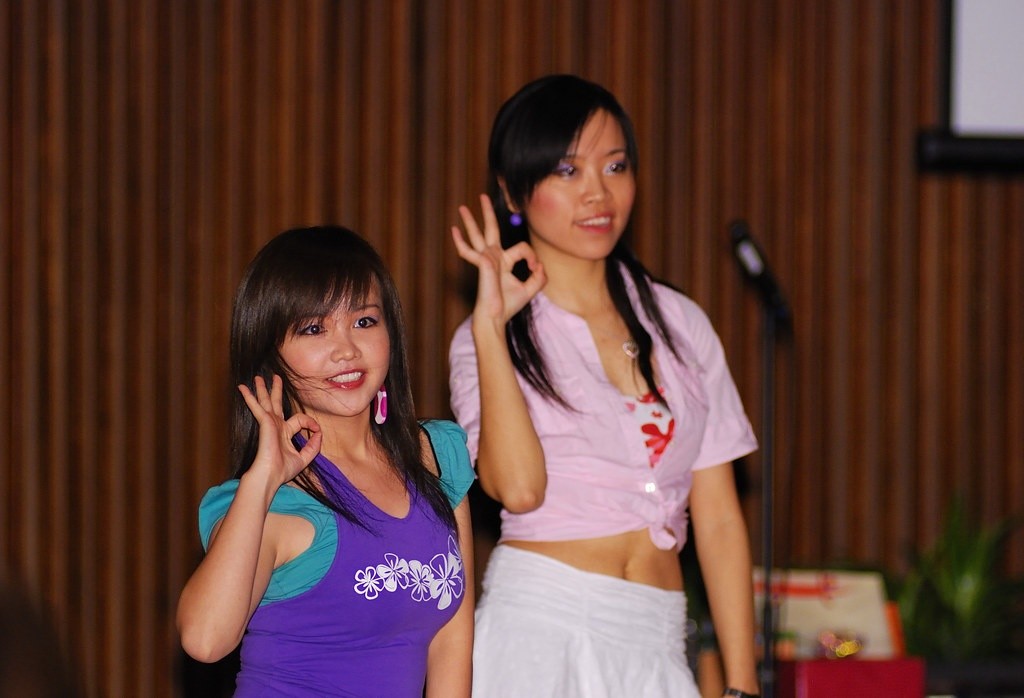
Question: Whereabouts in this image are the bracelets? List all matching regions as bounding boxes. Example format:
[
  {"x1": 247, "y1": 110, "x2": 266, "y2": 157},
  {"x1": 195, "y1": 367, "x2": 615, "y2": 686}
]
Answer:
[{"x1": 726, "y1": 687, "x2": 760, "y2": 698}]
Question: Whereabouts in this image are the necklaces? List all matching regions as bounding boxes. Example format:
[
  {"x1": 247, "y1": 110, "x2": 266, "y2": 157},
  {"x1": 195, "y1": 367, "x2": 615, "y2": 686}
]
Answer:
[{"x1": 592, "y1": 326, "x2": 639, "y2": 359}]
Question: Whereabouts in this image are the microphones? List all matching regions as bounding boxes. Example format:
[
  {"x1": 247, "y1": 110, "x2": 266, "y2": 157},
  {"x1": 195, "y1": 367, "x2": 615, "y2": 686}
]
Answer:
[{"x1": 724, "y1": 206, "x2": 797, "y2": 346}]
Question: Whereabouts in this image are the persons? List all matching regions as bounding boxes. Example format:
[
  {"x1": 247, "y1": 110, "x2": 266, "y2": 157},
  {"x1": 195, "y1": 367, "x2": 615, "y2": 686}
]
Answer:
[
  {"x1": 448, "y1": 73, "x2": 760, "y2": 698},
  {"x1": 175, "y1": 226, "x2": 476, "y2": 698}
]
